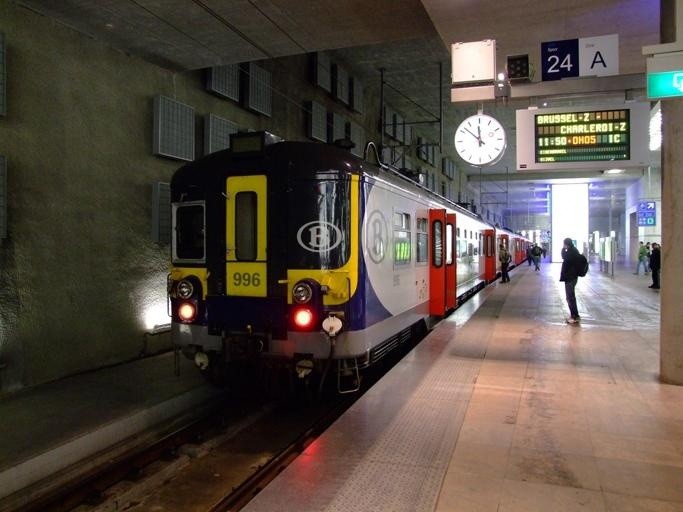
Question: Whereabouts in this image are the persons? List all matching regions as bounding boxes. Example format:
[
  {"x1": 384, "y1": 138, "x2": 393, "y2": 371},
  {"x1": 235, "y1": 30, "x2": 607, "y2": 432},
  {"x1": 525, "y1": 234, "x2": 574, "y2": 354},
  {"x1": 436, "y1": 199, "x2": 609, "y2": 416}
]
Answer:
[
  {"x1": 632, "y1": 241, "x2": 661, "y2": 289},
  {"x1": 559, "y1": 238, "x2": 581, "y2": 325},
  {"x1": 532, "y1": 243, "x2": 541, "y2": 271},
  {"x1": 498, "y1": 244, "x2": 512, "y2": 282},
  {"x1": 526, "y1": 246, "x2": 547, "y2": 266}
]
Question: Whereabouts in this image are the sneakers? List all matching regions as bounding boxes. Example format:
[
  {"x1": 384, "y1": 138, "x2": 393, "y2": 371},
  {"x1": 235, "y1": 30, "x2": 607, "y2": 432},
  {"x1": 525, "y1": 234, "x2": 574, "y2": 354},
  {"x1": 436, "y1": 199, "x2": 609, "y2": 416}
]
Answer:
[
  {"x1": 535, "y1": 268, "x2": 540, "y2": 271},
  {"x1": 566, "y1": 317, "x2": 580, "y2": 324},
  {"x1": 648, "y1": 284, "x2": 660, "y2": 289},
  {"x1": 500, "y1": 280, "x2": 510, "y2": 283}
]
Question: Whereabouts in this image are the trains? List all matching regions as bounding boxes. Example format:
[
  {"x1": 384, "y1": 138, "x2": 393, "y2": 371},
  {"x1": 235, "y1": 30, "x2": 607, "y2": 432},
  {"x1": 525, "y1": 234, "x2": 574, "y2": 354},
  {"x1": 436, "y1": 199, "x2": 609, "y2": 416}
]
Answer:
[{"x1": 162, "y1": 122, "x2": 536, "y2": 401}]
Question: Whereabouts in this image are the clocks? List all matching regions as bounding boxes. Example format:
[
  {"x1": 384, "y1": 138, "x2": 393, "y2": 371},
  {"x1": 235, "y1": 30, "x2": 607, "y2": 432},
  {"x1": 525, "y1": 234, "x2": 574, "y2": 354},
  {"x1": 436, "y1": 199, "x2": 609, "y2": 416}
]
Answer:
[{"x1": 453, "y1": 112, "x2": 507, "y2": 170}]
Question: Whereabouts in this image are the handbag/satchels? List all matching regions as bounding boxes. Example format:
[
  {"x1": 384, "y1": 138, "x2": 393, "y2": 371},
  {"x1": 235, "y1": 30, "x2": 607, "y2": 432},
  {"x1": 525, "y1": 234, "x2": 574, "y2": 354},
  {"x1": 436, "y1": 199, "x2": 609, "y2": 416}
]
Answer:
[{"x1": 576, "y1": 253, "x2": 588, "y2": 276}]
